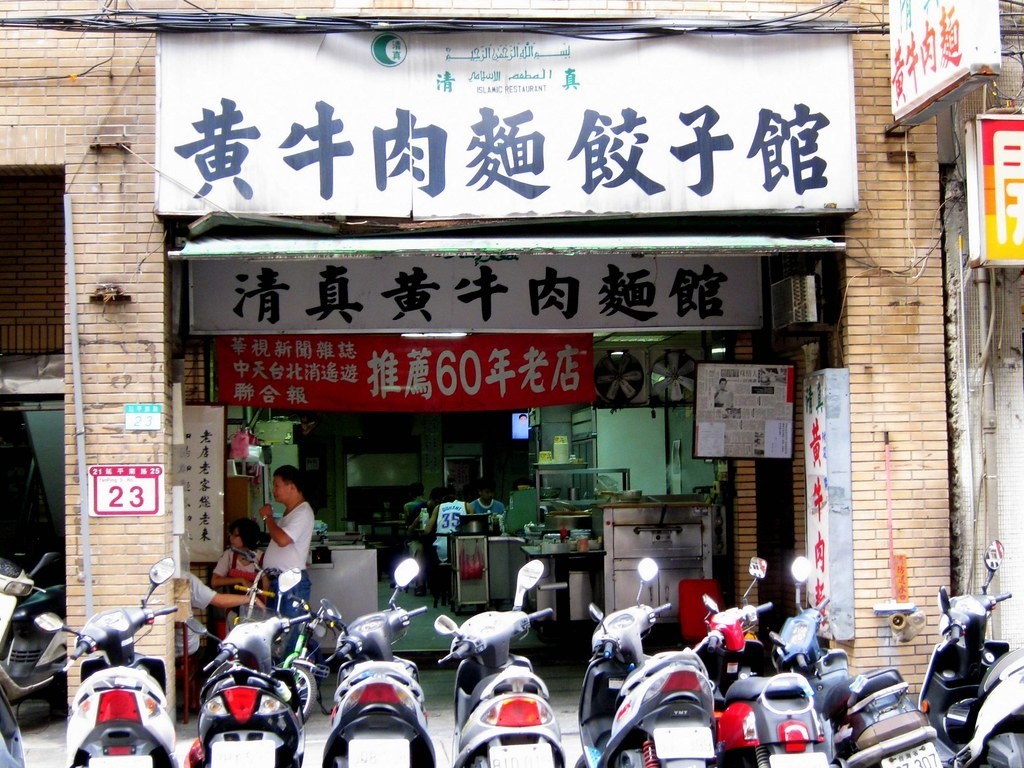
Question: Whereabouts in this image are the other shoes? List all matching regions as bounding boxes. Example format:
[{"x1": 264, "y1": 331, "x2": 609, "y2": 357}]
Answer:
[{"x1": 414, "y1": 584, "x2": 427, "y2": 596}]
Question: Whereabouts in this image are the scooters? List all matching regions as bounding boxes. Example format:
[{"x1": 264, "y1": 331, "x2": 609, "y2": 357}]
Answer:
[
  {"x1": 434, "y1": 559, "x2": 566, "y2": 768},
  {"x1": 0, "y1": 551, "x2": 67, "y2": 716},
  {"x1": 317, "y1": 558, "x2": 436, "y2": 768},
  {"x1": 769, "y1": 556, "x2": 943, "y2": 768},
  {"x1": 916, "y1": 539, "x2": 1024, "y2": 768},
  {"x1": 34, "y1": 556, "x2": 178, "y2": 768},
  {"x1": 694, "y1": 556, "x2": 830, "y2": 768},
  {"x1": 574, "y1": 557, "x2": 717, "y2": 768},
  {"x1": 184, "y1": 566, "x2": 312, "y2": 768}
]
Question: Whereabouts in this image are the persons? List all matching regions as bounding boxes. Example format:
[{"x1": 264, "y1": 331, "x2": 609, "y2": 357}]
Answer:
[
  {"x1": 713, "y1": 378, "x2": 732, "y2": 407},
  {"x1": 174, "y1": 569, "x2": 264, "y2": 706},
  {"x1": 258, "y1": 466, "x2": 314, "y2": 623},
  {"x1": 399, "y1": 478, "x2": 505, "y2": 595},
  {"x1": 519, "y1": 414, "x2": 527, "y2": 426},
  {"x1": 209, "y1": 517, "x2": 267, "y2": 637}
]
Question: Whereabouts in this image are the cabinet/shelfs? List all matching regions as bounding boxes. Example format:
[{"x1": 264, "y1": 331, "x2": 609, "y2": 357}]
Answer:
[
  {"x1": 536, "y1": 468, "x2": 631, "y2": 532},
  {"x1": 603, "y1": 505, "x2": 714, "y2": 624}
]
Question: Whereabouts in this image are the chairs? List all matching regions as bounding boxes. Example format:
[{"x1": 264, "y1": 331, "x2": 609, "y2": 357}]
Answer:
[
  {"x1": 175, "y1": 622, "x2": 201, "y2": 723},
  {"x1": 430, "y1": 532, "x2": 455, "y2": 608}
]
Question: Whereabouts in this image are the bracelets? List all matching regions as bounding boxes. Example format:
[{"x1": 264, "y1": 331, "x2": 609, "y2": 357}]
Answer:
[{"x1": 262, "y1": 515, "x2": 271, "y2": 521}]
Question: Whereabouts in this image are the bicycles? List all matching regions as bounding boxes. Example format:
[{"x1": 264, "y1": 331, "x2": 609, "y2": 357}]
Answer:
[
  {"x1": 275, "y1": 594, "x2": 348, "y2": 725},
  {"x1": 232, "y1": 546, "x2": 296, "y2": 666}
]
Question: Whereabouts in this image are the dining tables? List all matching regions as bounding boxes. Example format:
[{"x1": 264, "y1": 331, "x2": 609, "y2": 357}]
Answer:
[{"x1": 341, "y1": 515, "x2": 406, "y2": 582}]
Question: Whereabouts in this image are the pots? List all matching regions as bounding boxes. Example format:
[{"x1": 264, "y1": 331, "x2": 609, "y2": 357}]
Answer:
[{"x1": 455, "y1": 511, "x2": 494, "y2": 534}]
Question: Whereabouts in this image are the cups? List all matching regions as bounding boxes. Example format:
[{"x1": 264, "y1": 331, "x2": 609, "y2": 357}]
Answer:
[
  {"x1": 567, "y1": 487, "x2": 580, "y2": 500},
  {"x1": 554, "y1": 443, "x2": 568, "y2": 463}
]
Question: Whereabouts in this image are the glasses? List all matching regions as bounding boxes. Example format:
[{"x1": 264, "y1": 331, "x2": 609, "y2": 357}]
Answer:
[{"x1": 230, "y1": 532, "x2": 240, "y2": 537}]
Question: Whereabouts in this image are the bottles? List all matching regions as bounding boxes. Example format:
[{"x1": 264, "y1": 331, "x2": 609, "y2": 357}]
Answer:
[{"x1": 560, "y1": 525, "x2": 566, "y2": 544}]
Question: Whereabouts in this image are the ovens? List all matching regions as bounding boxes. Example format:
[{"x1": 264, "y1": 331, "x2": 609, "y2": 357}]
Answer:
[{"x1": 591, "y1": 498, "x2": 713, "y2": 623}]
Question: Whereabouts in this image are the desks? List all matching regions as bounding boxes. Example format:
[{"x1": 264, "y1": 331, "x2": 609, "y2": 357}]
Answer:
[{"x1": 520, "y1": 539, "x2": 604, "y2": 638}]
